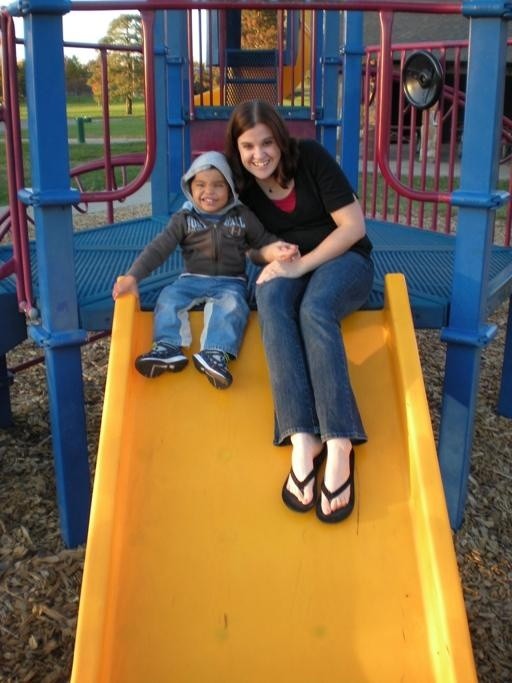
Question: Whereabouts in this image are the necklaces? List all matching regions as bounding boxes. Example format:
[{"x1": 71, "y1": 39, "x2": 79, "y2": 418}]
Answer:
[{"x1": 265, "y1": 180, "x2": 278, "y2": 192}]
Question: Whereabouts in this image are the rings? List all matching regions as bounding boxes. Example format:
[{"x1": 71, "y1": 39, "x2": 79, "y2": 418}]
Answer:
[{"x1": 271, "y1": 269, "x2": 276, "y2": 274}]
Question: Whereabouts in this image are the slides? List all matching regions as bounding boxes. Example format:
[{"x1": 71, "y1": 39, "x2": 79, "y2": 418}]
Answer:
[
  {"x1": 193, "y1": 8, "x2": 310, "y2": 106},
  {"x1": 68, "y1": 273, "x2": 479, "y2": 683}
]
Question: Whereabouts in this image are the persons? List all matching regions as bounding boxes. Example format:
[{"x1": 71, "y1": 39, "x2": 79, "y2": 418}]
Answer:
[
  {"x1": 222, "y1": 98, "x2": 377, "y2": 525},
  {"x1": 110, "y1": 150, "x2": 301, "y2": 391}
]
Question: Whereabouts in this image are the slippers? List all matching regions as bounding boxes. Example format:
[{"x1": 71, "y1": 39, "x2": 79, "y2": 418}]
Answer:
[
  {"x1": 284, "y1": 459, "x2": 318, "y2": 512},
  {"x1": 318, "y1": 450, "x2": 355, "y2": 524}
]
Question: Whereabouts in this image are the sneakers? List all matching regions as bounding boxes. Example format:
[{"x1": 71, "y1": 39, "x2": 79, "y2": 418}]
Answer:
[
  {"x1": 194, "y1": 346, "x2": 232, "y2": 389},
  {"x1": 136, "y1": 341, "x2": 190, "y2": 378}
]
